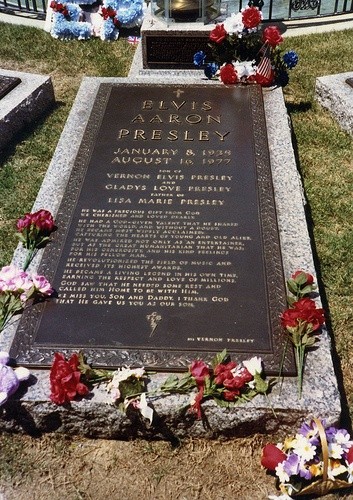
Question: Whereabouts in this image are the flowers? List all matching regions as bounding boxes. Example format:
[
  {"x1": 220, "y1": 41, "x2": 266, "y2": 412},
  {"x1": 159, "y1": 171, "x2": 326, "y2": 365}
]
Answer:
[
  {"x1": 50, "y1": 0, "x2": 300, "y2": 86},
  {"x1": 0, "y1": 209, "x2": 353, "y2": 495}
]
